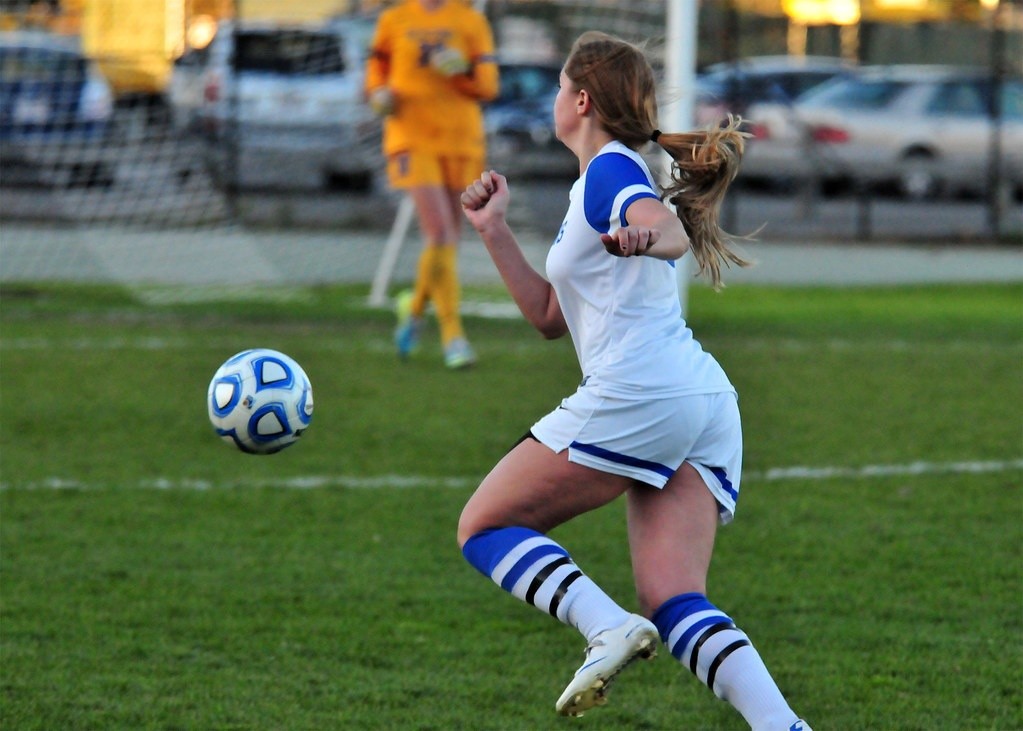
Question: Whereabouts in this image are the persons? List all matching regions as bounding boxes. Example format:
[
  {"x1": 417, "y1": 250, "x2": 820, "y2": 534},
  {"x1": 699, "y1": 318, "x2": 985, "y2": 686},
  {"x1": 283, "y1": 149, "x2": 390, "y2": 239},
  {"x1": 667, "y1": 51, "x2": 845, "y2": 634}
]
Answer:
[
  {"x1": 368, "y1": 0, "x2": 501, "y2": 367},
  {"x1": 454, "y1": 29, "x2": 820, "y2": 731}
]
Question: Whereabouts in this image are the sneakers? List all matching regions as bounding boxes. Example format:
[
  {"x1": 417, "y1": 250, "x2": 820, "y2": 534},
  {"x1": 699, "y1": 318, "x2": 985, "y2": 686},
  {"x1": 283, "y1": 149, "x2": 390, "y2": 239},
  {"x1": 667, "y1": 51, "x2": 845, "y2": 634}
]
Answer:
[
  {"x1": 552, "y1": 611, "x2": 661, "y2": 718},
  {"x1": 390, "y1": 286, "x2": 426, "y2": 364},
  {"x1": 442, "y1": 336, "x2": 478, "y2": 369}
]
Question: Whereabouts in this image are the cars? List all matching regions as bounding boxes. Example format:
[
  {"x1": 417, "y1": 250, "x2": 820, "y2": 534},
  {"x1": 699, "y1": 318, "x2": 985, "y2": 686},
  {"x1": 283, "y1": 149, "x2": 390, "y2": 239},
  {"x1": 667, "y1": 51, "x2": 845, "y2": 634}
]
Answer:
[
  {"x1": 483, "y1": 62, "x2": 579, "y2": 158},
  {"x1": 690, "y1": 55, "x2": 847, "y2": 188},
  {"x1": 781, "y1": 58, "x2": 1022, "y2": 197},
  {"x1": 184, "y1": 21, "x2": 373, "y2": 194},
  {"x1": 0, "y1": 37, "x2": 120, "y2": 196}
]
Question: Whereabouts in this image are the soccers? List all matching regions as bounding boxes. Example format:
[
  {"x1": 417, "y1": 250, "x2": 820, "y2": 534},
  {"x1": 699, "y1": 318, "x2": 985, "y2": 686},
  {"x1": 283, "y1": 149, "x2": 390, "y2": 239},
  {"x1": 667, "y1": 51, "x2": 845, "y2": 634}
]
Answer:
[{"x1": 206, "y1": 349, "x2": 313, "y2": 454}]
